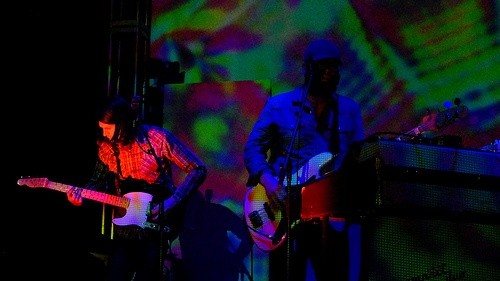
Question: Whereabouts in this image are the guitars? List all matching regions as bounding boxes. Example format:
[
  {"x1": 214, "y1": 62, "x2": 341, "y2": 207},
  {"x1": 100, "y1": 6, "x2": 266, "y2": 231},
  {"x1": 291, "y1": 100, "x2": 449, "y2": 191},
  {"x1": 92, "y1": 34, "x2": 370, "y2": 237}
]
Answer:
[
  {"x1": 243, "y1": 97, "x2": 474, "y2": 253},
  {"x1": 17, "y1": 177, "x2": 180, "y2": 238}
]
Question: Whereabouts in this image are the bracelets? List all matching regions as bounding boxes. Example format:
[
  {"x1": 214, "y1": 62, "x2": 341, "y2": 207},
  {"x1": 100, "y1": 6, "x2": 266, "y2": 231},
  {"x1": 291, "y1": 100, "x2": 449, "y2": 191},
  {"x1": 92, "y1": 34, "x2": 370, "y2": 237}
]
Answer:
[{"x1": 259, "y1": 173, "x2": 272, "y2": 183}]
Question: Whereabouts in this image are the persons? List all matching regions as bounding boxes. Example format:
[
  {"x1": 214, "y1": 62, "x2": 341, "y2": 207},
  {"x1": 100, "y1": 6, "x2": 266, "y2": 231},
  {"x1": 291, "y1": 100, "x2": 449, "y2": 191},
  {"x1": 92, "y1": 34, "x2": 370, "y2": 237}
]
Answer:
[
  {"x1": 65, "y1": 96, "x2": 208, "y2": 281},
  {"x1": 244, "y1": 38, "x2": 390, "y2": 281}
]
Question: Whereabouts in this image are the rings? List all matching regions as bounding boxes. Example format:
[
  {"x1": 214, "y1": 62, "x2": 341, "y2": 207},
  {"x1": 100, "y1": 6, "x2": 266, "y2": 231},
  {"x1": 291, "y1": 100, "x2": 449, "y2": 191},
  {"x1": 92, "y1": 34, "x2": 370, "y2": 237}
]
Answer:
[{"x1": 73, "y1": 190, "x2": 78, "y2": 194}]
